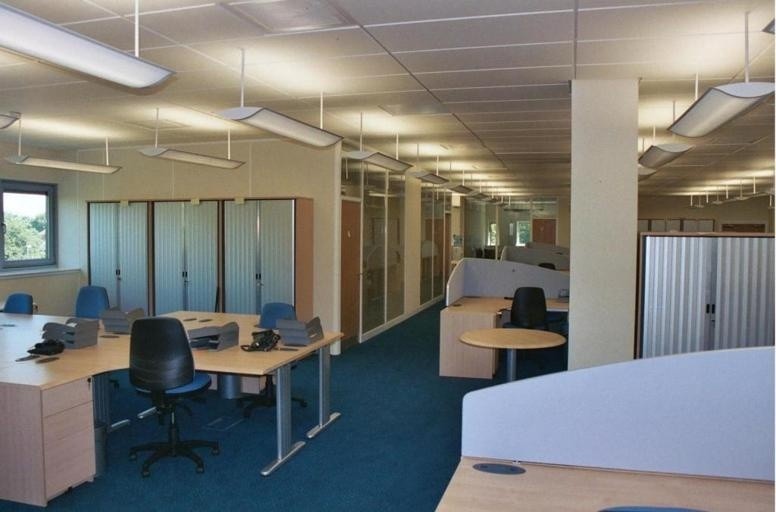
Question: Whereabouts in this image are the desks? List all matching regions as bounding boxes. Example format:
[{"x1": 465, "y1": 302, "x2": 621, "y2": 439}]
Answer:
[
  {"x1": 434, "y1": 457, "x2": 774, "y2": 511},
  {"x1": 0, "y1": 300, "x2": 345, "y2": 508},
  {"x1": 439, "y1": 296, "x2": 569, "y2": 379}
]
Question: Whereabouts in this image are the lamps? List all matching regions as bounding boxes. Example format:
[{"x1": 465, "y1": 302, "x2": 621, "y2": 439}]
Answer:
[
  {"x1": 638, "y1": 10, "x2": 775, "y2": 182},
  {"x1": 344, "y1": 113, "x2": 498, "y2": 203}
]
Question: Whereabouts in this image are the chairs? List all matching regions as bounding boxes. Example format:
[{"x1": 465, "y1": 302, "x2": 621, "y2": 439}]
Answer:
[{"x1": 503, "y1": 287, "x2": 551, "y2": 370}]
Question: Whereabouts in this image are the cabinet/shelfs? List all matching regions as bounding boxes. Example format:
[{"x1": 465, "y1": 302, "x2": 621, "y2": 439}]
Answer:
[
  {"x1": 88, "y1": 198, "x2": 314, "y2": 324},
  {"x1": 635, "y1": 219, "x2": 775, "y2": 359}
]
